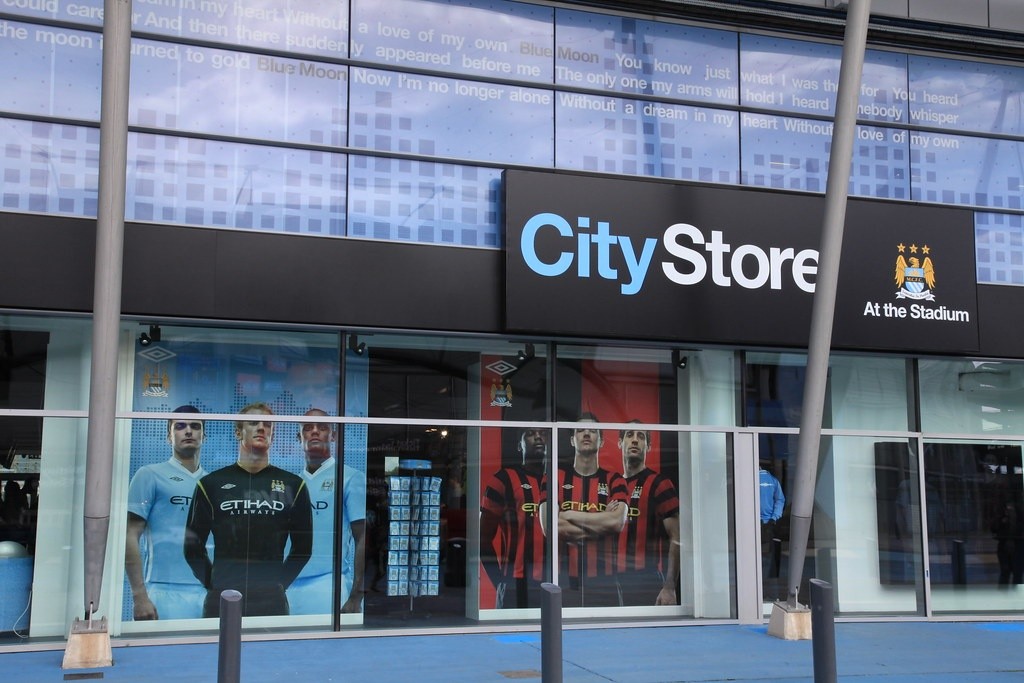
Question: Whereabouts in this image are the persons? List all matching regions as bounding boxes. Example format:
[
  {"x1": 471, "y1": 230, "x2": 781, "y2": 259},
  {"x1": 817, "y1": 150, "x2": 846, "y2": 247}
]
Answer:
[
  {"x1": 0, "y1": 476, "x2": 39, "y2": 553},
  {"x1": 284, "y1": 409, "x2": 364, "y2": 615},
  {"x1": 760, "y1": 463, "x2": 786, "y2": 599},
  {"x1": 539, "y1": 411, "x2": 630, "y2": 607},
  {"x1": 183, "y1": 404, "x2": 313, "y2": 618},
  {"x1": 480, "y1": 425, "x2": 554, "y2": 609},
  {"x1": 877, "y1": 468, "x2": 1024, "y2": 592},
  {"x1": 124, "y1": 405, "x2": 214, "y2": 622},
  {"x1": 617, "y1": 419, "x2": 680, "y2": 607}
]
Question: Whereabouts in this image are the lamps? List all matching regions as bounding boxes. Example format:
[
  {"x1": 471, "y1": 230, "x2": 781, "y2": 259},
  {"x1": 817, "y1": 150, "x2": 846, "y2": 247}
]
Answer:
[
  {"x1": 140, "y1": 333, "x2": 151, "y2": 346},
  {"x1": 518, "y1": 350, "x2": 526, "y2": 361},
  {"x1": 678, "y1": 356, "x2": 688, "y2": 369},
  {"x1": 355, "y1": 342, "x2": 366, "y2": 356}
]
